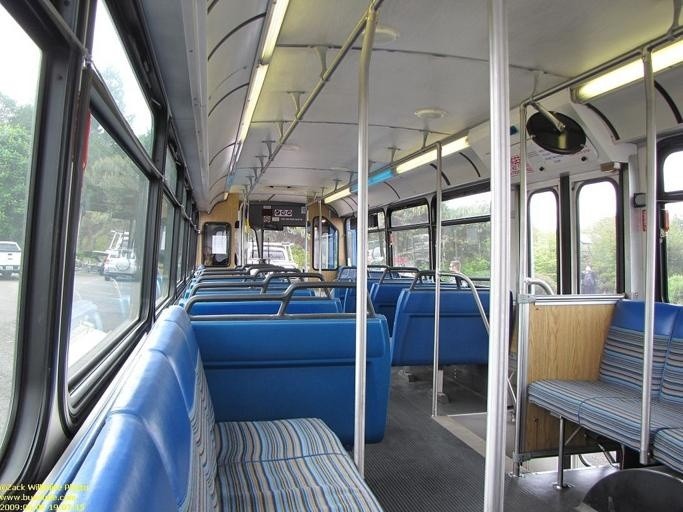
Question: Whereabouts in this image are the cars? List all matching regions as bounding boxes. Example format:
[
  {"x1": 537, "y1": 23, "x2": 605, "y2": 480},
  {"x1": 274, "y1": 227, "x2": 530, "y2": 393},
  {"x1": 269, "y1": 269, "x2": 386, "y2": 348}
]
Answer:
[{"x1": 246, "y1": 241, "x2": 297, "y2": 270}]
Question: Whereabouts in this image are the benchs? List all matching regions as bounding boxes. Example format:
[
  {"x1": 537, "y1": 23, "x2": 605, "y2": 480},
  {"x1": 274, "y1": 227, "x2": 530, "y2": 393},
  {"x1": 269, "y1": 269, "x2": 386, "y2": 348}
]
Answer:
[
  {"x1": 21, "y1": 264, "x2": 493, "y2": 512},
  {"x1": 527, "y1": 299, "x2": 683, "y2": 493}
]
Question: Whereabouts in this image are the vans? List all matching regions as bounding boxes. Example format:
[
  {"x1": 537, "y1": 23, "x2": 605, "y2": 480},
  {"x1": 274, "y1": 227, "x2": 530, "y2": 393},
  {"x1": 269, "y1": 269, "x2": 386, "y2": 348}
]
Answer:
[{"x1": 116, "y1": 237, "x2": 129, "y2": 248}]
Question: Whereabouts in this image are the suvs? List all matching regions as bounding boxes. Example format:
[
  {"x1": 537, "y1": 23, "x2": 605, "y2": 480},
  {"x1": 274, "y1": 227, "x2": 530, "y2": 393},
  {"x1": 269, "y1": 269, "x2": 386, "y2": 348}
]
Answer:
[{"x1": 103, "y1": 249, "x2": 141, "y2": 281}]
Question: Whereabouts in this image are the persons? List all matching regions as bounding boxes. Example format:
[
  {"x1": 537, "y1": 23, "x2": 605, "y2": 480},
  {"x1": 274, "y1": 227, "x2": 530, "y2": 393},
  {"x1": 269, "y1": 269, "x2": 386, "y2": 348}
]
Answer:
[
  {"x1": 580, "y1": 265, "x2": 596, "y2": 295},
  {"x1": 446, "y1": 259, "x2": 469, "y2": 286}
]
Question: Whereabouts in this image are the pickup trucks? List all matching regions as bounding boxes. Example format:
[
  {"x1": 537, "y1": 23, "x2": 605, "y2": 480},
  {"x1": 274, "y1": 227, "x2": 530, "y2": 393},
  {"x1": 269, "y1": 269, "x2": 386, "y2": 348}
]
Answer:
[{"x1": 0, "y1": 241, "x2": 21, "y2": 278}]
